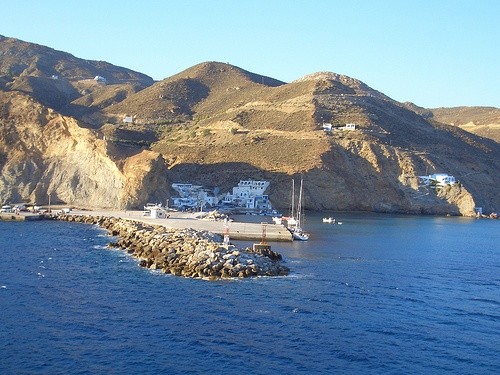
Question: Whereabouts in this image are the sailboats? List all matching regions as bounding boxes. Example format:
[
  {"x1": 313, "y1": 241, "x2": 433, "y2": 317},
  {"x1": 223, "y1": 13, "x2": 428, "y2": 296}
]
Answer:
[{"x1": 283, "y1": 177, "x2": 310, "y2": 240}]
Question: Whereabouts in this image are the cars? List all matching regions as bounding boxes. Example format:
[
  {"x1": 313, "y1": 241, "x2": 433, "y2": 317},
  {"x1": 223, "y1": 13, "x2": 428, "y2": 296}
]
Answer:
[{"x1": 1, "y1": 204, "x2": 71, "y2": 217}]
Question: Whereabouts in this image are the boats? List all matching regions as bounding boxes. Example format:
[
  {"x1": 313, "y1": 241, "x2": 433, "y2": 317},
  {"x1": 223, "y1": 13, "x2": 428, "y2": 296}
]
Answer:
[{"x1": 324, "y1": 217, "x2": 334, "y2": 223}]
